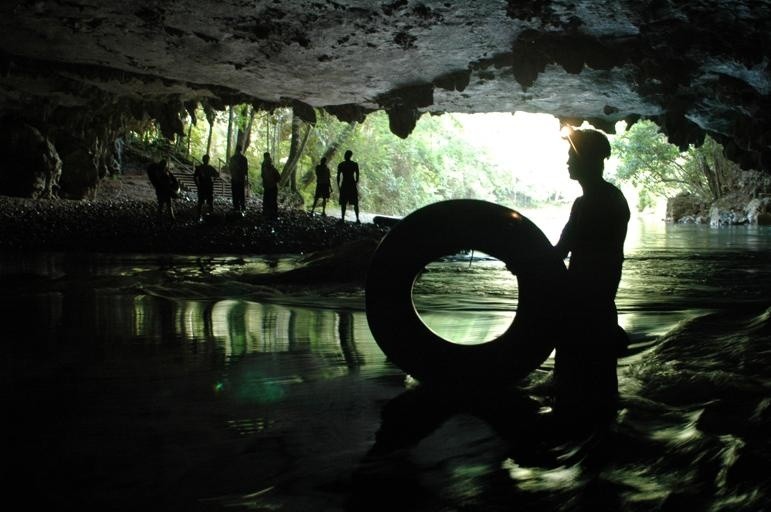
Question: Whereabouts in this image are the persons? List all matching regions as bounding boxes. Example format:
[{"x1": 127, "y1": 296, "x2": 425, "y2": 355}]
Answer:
[
  {"x1": 229, "y1": 144, "x2": 249, "y2": 212},
  {"x1": 260, "y1": 151, "x2": 283, "y2": 218},
  {"x1": 148, "y1": 158, "x2": 182, "y2": 221},
  {"x1": 193, "y1": 153, "x2": 220, "y2": 218},
  {"x1": 310, "y1": 157, "x2": 334, "y2": 216},
  {"x1": 505, "y1": 127, "x2": 633, "y2": 427},
  {"x1": 337, "y1": 150, "x2": 363, "y2": 224}
]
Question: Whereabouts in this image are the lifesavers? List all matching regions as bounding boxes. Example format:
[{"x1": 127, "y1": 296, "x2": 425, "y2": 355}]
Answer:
[{"x1": 363, "y1": 199, "x2": 568, "y2": 391}]
